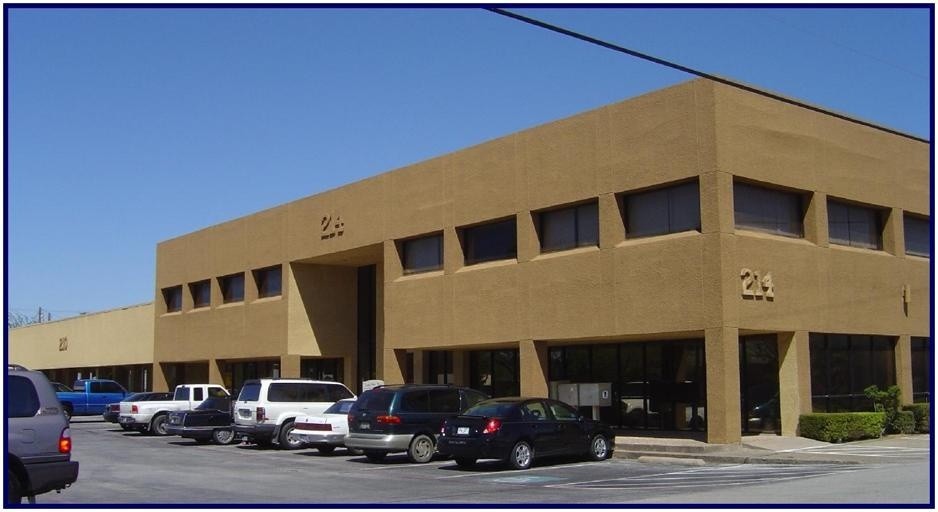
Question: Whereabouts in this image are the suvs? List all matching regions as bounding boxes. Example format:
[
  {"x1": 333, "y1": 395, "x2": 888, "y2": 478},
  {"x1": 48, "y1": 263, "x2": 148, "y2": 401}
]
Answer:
[
  {"x1": 233, "y1": 376, "x2": 357, "y2": 450},
  {"x1": 5, "y1": 362, "x2": 79, "y2": 506}
]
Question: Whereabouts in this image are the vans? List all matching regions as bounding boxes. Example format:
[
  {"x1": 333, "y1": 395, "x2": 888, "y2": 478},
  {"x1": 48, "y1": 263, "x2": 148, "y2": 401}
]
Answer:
[{"x1": 343, "y1": 384, "x2": 492, "y2": 462}]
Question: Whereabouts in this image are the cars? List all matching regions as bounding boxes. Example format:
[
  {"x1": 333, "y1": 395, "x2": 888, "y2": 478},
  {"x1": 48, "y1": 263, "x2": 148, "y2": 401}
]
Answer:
[
  {"x1": 290, "y1": 396, "x2": 356, "y2": 455},
  {"x1": 47, "y1": 380, "x2": 74, "y2": 392},
  {"x1": 104, "y1": 390, "x2": 173, "y2": 422},
  {"x1": 167, "y1": 395, "x2": 240, "y2": 445},
  {"x1": 444, "y1": 396, "x2": 615, "y2": 468}
]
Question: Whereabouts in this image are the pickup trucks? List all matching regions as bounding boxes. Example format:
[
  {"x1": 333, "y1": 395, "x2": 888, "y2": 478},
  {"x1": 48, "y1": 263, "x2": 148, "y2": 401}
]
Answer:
[
  {"x1": 53, "y1": 375, "x2": 155, "y2": 422},
  {"x1": 119, "y1": 383, "x2": 231, "y2": 437}
]
